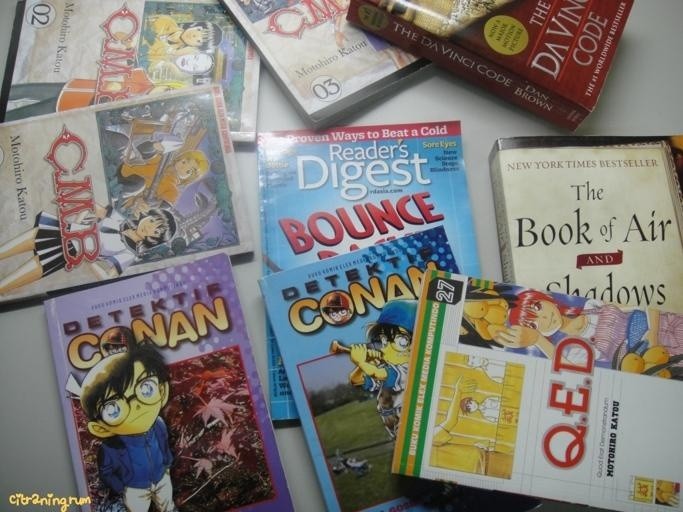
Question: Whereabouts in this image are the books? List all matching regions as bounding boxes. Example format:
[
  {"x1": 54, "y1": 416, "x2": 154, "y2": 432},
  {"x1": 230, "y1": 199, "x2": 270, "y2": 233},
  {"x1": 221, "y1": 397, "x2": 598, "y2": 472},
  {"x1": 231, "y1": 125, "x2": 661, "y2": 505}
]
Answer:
[
  {"x1": 486, "y1": 133, "x2": 683, "y2": 312},
  {"x1": 345, "y1": 0, "x2": 635, "y2": 131},
  {"x1": 44, "y1": 252, "x2": 296, "y2": 511},
  {"x1": 255, "y1": 119, "x2": 481, "y2": 423},
  {"x1": 4, "y1": 0, "x2": 260, "y2": 147},
  {"x1": 256, "y1": 221, "x2": 462, "y2": 511},
  {"x1": 0, "y1": 82, "x2": 254, "y2": 307},
  {"x1": 390, "y1": 266, "x2": 682, "y2": 512},
  {"x1": 220, "y1": 0, "x2": 435, "y2": 133}
]
